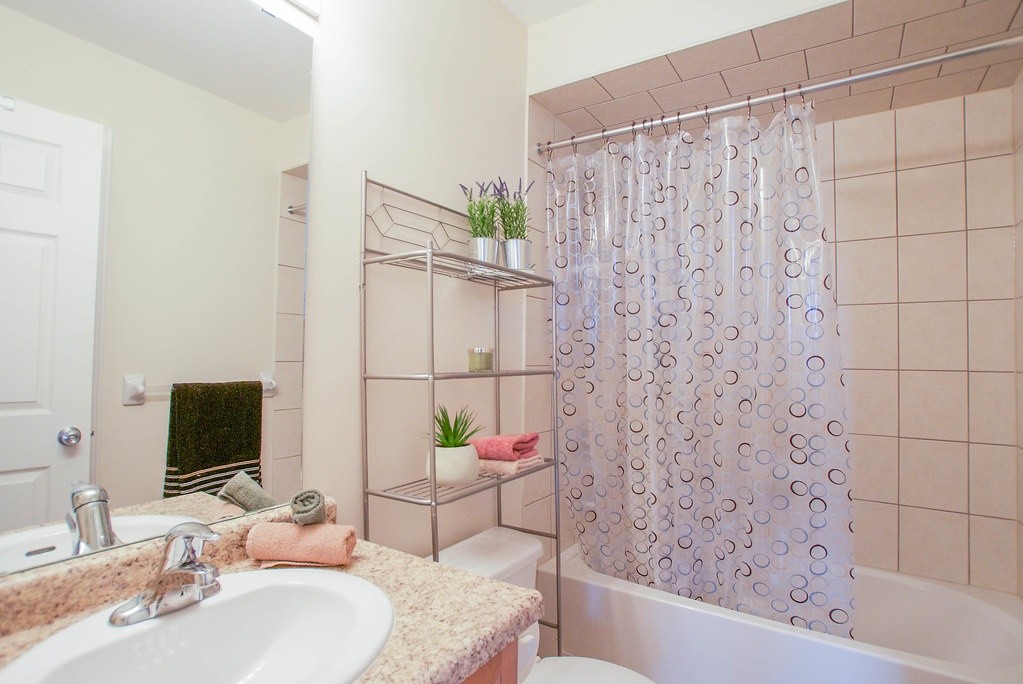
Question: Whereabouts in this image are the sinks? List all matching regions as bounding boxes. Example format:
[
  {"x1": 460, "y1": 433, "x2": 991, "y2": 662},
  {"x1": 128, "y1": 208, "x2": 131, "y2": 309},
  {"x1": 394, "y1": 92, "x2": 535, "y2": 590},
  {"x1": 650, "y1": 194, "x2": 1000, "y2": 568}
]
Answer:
[
  {"x1": 1, "y1": 514, "x2": 205, "y2": 576},
  {"x1": 1, "y1": 566, "x2": 395, "y2": 684}
]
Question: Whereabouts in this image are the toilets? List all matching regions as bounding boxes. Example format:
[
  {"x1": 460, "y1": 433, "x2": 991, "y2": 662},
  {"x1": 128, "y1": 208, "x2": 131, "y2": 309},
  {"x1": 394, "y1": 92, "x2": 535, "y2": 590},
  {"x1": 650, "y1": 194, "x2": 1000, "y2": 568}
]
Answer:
[{"x1": 423, "y1": 524, "x2": 658, "y2": 684}]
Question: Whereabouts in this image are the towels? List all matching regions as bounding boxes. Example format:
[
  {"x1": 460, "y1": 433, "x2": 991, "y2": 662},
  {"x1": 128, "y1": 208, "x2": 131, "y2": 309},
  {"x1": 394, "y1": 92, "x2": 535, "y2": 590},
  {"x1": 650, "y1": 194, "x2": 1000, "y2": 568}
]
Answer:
[
  {"x1": 162, "y1": 381, "x2": 263, "y2": 497},
  {"x1": 289, "y1": 488, "x2": 326, "y2": 526},
  {"x1": 464, "y1": 432, "x2": 544, "y2": 475},
  {"x1": 218, "y1": 470, "x2": 279, "y2": 512},
  {"x1": 244, "y1": 521, "x2": 358, "y2": 569},
  {"x1": 214, "y1": 501, "x2": 246, "y2": 520}
]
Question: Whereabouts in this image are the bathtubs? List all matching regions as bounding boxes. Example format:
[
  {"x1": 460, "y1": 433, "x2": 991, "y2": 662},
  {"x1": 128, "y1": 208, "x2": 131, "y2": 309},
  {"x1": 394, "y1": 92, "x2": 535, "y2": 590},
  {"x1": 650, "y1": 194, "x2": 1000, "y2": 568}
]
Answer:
[{"x1": 537, "y1": 544, "x2": 1023, "y2": 683}]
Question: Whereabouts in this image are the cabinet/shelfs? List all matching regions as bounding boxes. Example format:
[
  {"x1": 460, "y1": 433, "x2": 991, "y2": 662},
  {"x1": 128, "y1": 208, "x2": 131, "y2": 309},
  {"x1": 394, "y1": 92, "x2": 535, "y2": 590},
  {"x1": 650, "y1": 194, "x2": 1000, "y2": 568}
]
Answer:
[{"x1": 362, "y1": 170, "x2": 564, "y2": 659}]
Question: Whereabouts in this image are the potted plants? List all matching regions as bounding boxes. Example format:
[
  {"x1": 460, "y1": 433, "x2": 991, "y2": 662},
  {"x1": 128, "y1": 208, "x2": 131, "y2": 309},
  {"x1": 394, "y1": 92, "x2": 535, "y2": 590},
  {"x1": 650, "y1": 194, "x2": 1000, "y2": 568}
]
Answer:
[
  {"x1": 426, "y1": 403, "x2": 487, "y2": 487},
  {"x1": 491, "y1": 175, "x2": 535, "y2": 281},
  {"x1": 459, "y1": 180, "x2": 507, "y2": 280}
]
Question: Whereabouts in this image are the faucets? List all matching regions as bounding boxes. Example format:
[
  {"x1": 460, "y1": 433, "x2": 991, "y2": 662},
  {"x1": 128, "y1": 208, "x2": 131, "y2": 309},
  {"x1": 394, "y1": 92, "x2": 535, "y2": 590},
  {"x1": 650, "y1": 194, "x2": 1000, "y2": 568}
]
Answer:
[
  {"x1": 66, "y1": 478, "x2": 125, "y2": 558},
  {"x1": 108, "y1": 522, "x2": 222, "y2": 625}
]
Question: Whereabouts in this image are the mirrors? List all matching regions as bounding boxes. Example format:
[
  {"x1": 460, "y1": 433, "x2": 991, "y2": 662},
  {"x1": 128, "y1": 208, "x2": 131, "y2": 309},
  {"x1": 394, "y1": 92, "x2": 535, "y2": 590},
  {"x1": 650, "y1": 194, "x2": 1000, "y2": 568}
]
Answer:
[{"x1": 2, "y1": 0, "x2": 312, "y2": 574}]
